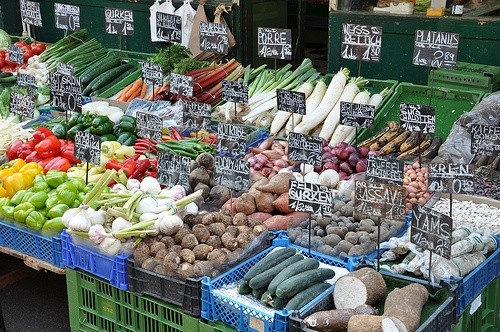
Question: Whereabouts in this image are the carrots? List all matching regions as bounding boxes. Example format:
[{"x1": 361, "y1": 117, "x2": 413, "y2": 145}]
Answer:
[{"x1": 117, "y1": 43, "x2": 211, "y2": 105}]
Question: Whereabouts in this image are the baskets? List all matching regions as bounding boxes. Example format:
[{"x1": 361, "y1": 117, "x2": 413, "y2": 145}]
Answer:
[{"x1": 1, "y1": 33, "x2": 500, "y2": 332}]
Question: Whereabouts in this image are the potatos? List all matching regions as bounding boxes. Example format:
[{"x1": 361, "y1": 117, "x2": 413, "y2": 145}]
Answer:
[
  {"x1": 287, "y1": 198, "x2": 407, "y2": 262},
  {"x1": 221, "y1": 172, "x2": 312, "y2": 233}
]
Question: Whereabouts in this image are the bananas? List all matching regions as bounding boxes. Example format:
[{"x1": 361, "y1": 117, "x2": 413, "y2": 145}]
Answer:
[{"x1": 356, "y1": 120, "x2": 443, "y2": 159}]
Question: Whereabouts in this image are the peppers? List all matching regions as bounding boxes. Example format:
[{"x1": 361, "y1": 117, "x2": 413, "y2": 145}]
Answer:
[{"x1": 0, "y1": 111, "x2": 216, "y2": 234}]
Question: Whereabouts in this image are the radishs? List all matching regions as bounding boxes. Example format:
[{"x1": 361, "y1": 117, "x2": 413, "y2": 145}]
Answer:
[{"x1": 269, "y1": 67, "x2": 394, "y2": 146}]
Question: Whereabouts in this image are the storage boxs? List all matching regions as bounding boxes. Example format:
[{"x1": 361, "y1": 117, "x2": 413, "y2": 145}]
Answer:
[{"x1": 0, "y1": 33, "x2": 500, "y2": 332}]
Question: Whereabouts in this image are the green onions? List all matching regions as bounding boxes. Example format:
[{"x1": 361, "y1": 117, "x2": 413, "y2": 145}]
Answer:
[
  {"x1": 208, "y1": 57, "x2": 321, "y2": 128},
  {"x1": 25, "y1": 28, "x2": 106, "y2": 89}
]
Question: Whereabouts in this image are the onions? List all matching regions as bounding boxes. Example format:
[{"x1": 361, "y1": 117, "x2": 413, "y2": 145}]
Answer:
[{"x1": 314, "y1": 137, "x2": 385, "y2": 182}]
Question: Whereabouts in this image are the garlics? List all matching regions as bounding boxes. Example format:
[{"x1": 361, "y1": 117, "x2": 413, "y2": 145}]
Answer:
[{"x1": 62, "y1": 175, "x2": 203, "y2": 256}]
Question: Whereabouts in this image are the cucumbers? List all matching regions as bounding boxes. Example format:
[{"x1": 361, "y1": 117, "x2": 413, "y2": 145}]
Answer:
[
  {"x1": 237, "y1": 245, "x2": 335, "y2": 314},
  {"x1": 74, "y1": 52, "x2": 139, "y2": 98}
]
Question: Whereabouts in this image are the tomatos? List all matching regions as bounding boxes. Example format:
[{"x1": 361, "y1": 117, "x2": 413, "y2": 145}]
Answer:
[{"x1": 0, "y1": 39, "x2": 46, "y2": 74}]
